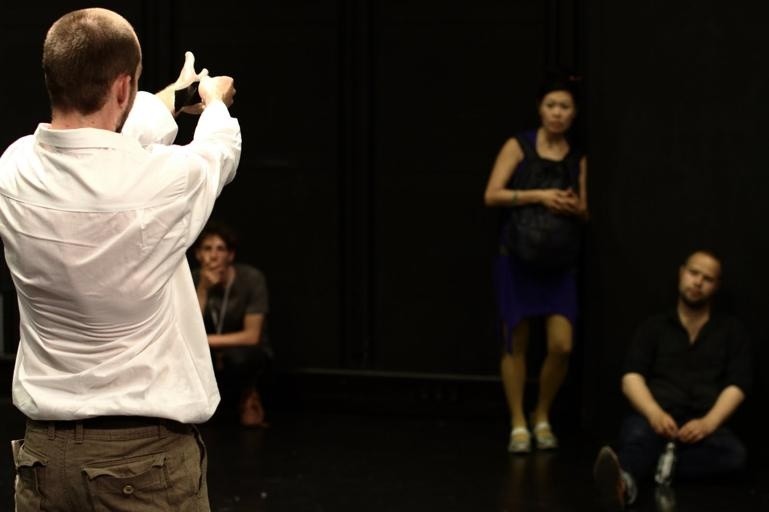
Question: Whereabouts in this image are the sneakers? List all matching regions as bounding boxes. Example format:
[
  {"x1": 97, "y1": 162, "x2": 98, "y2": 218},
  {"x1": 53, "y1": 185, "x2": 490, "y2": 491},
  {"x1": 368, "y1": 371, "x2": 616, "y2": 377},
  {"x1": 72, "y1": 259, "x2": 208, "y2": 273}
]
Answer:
[
  {"x1": 508, "y1": 413, "x2": 558, "y2": 454},
  {"x1": 593, "y1": 443, "x2": 675, "y2": 511}
]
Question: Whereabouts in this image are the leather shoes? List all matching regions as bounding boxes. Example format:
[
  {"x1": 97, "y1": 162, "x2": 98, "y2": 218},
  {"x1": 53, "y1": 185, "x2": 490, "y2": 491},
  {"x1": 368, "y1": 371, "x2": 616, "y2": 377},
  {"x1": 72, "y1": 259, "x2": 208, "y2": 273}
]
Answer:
[{"x1": 241, "y1": 389, "x2": 263, "y2": 427}]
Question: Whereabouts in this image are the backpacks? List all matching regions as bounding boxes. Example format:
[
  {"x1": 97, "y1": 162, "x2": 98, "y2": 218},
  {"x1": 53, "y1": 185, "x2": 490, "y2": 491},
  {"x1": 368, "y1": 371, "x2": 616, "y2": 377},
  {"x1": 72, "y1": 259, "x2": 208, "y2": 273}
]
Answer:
[{"x1": 510, "y1": 135, "x2": 580, "y2": 265}]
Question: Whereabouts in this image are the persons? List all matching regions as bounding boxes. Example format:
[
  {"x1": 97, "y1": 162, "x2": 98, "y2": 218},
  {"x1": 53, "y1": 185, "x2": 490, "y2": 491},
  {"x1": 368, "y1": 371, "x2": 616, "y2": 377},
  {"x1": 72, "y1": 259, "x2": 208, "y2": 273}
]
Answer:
[
  {"x1": 2, "y1": 7, "x2": 244, "y2": 512},
  {"x1": 483, "y1": 89, "x2": 587, "y2": 453},
  {"x1": 185, "y1": 222, "x2": 267, "y2": 510},
  {"x1": 594, "y1": 244, "x2": 746, "y2": 512}
]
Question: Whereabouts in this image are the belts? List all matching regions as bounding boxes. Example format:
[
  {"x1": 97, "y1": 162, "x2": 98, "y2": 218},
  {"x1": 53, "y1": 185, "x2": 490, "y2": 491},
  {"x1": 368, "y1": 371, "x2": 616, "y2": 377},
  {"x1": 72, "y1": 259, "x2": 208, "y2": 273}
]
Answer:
[{"x1": 28, "y1": 416, "x2": 178, "y2": 431}]
